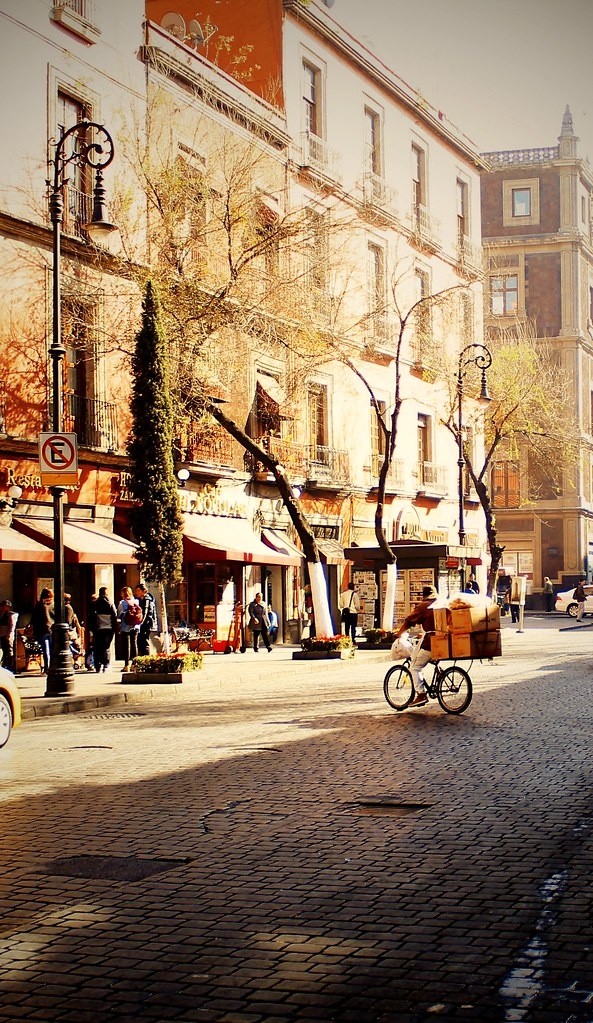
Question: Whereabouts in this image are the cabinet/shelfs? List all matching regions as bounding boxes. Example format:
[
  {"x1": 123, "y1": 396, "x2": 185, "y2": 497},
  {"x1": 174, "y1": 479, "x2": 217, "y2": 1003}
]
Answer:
[
  {"x1": 407, "y1": 568, "x2": 435, "y2": 615},
  {"x1": 380, "y1": 569, "x2": 407, "y2": 628}
]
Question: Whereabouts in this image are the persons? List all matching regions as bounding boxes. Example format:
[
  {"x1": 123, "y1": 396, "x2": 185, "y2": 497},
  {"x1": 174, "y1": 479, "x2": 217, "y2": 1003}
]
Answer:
[
  {"x1": 465, "y1": 574, "x2": 480, "y2": 594},
  {"x1": 576, "y1": 580, "x2": 588, "y2": 622},
  {"x1": 83, "y1": 587, "x2": 119, "y2": 673},
  {"x1": 545, "y1": 577, "x2": 553, "y2": 612},
  {"x1": 506, "y1": 580, "x2": 520, "y2": 623},
  {"x1": 0, "y1": 600, "x2": 19, "y2": 670},
  {"x1": 340, "y1": 583, "x2": 361, "y2": 644},
  {"x1": 394, "y1": 587, "x2": 439, "y2": 707},
  {"x1": 118, "y1": 587, "x2": 139, "y2": 672},
  {"x1": 267, "y1": 604, "x2": 278, "y2": 644},
  {"x1": 63, "y1": 594, "x2": 73, "y2": 626},
  {"x1": 32, "y1": 589, "x2": 54, "y2": 676},
  {"x1": 248, "y1": 593, "x2": 273, "y2": 652},
  {"x1": 135, "y1": 584, "x2": 157, "y2": 655}
]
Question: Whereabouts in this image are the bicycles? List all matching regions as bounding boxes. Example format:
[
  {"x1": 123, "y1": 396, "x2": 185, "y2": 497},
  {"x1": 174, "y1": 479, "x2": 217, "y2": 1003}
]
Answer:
[{"x1": 382, "y1": 630, "x2": 473, "y2": 715}]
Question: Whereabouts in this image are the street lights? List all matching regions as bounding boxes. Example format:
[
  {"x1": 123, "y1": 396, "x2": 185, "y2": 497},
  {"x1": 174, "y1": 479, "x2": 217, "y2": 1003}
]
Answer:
[
  {"x1": 456, "y1": 341, "x2": 494, "y2": 595},
  {"x1": 40, "y1": 120, "x2": 120, "y2": 698}
]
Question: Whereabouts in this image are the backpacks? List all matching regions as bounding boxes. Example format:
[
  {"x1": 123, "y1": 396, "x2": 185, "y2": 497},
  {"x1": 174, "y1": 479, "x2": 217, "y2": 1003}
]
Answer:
[{"x1": 125, "y1": 597, "x2": 145, "y2": 625}]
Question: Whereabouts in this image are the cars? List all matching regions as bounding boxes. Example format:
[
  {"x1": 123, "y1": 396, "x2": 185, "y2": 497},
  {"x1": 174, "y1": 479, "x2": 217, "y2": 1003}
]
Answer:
[{"x1": 554, "y1": 583, "x2": 593, "y2": 617}]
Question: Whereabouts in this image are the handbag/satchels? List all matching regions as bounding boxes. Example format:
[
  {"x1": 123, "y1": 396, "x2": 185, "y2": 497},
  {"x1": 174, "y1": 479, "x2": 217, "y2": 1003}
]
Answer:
[
  {"x1": 340, "y1": 608, "x2": 350, "y2": 623},
  {"x1": 391, "y1": 633, "x2": 414, "y2": 661}
]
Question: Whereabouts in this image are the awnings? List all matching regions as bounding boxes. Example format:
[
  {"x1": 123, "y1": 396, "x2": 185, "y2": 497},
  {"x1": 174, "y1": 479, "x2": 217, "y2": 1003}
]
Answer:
[
  {"x1": 316, "y1": 539, "x2": 354, "y2": 565},
  {"x1": 12, "y1": 518, "x2": 148, "y2": 564},
  {"x1": 0, "y1": 526, "x2": 54, "y2": 562},
  {"x1": 179, "y1": 514, "x2": 306, "y2": 565},
  {"x1": 179, "y1": 353, "x2": 232, "y2": 404},
  {"x1": 258, "y1": 374, "x2": 300, "y2": 421}
]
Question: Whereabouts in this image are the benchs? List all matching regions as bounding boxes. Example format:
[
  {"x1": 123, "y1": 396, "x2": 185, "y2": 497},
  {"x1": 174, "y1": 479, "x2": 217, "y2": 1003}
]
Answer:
[
  {"x1": 19, "y1": 624, "x2": 47, "y2": 673},
  {"x1": 173, "y1": 626, "x2": 214, "y2": 654}
]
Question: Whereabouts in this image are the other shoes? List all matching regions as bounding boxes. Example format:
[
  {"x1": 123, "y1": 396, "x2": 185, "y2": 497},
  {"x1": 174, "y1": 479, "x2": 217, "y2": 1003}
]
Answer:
[
  {"x1": 121, "y1": 665, "x2": 129, "y2": 671},
  {"x1": 255, "y1": 648, "x2": 259, "y2": 652},
  {"x1": 268, "y1": 646, "x2": 272, "y2": 651},
  {"x1": 407, "y1": 693, "x2": 428, "y2": 707},
  {"x1": 39, "y1": 673, "x2": 48, "y2": 677}
]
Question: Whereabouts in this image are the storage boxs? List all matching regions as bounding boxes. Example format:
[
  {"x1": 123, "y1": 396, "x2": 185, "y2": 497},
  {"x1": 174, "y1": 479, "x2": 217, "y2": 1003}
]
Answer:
[
  {"x1": 352, "y1": 572, "x2": 378, "y2": 630},
  {"x1": 430, "y1": 605, "x2": 503, "y2": 659}
]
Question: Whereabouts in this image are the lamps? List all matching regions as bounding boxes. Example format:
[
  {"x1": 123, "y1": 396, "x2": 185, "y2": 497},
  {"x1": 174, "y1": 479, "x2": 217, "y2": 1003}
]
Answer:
[
  {"x1": 547, "y1": 546, "x2": 563, "y2": 560},
  {"x1": 0, "y1": 486, "x2": 23, "y2": 508},
  {"x1": 174, "y1": 468, "x2": 190, "y2": 487}
]
